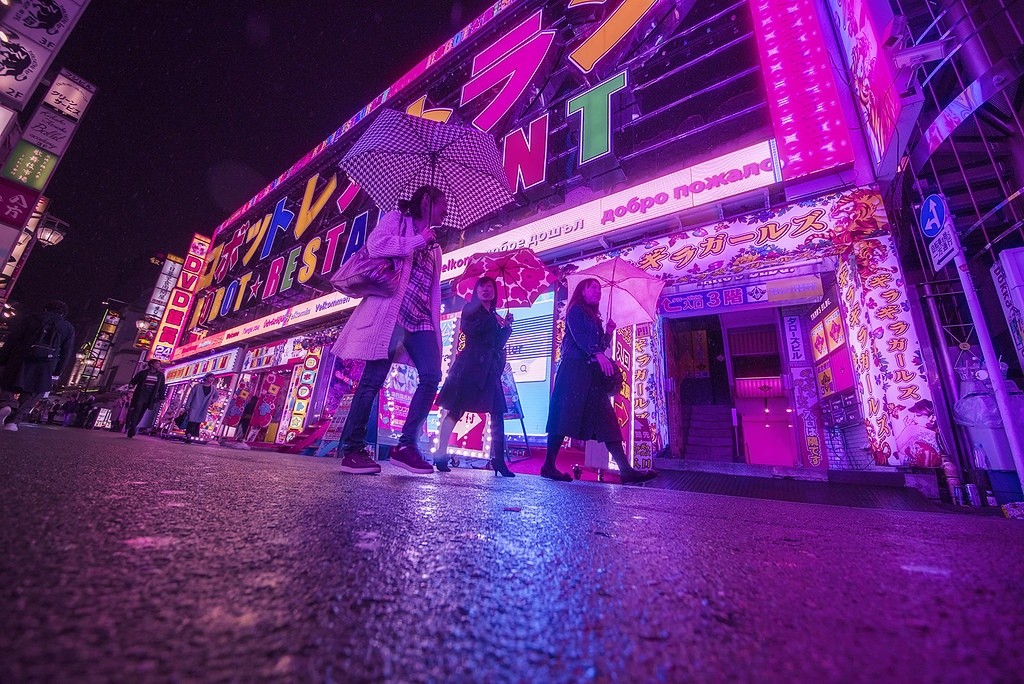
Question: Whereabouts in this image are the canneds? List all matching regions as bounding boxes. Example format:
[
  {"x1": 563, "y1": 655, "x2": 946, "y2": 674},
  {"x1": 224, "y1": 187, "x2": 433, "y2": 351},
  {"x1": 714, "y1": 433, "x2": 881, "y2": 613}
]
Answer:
[{"x1": 953, "y1": 483, "x2": 983, "y2": 507}]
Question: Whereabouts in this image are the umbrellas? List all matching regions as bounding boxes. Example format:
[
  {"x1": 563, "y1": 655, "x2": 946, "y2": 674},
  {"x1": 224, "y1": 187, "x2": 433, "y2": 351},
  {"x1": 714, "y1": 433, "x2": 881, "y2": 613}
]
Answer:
[
  {"x1": 338, "y1": 108, "x2": 517, "y2": 231},
  {"x1": 452, "y1": 248, "x2": 557, "y2": 321},
  {"x1": 565, "y1": 256, "x2": 665, "y2": 332}
]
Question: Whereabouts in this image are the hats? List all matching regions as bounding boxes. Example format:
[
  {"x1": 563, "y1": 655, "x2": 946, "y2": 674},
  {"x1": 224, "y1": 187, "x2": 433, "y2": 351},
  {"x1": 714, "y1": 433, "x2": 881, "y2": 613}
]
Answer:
[{"x1": 203, "y1": 372, "x2": 214, "y2": 381}]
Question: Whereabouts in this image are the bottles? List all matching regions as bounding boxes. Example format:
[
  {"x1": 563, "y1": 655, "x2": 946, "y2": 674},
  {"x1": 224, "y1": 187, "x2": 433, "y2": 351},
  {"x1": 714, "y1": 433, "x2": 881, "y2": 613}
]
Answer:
[
  {"x1": 973, "y1": 442, "x2": 992, "y2": 471},
  {"x1": 986, "y1": 490, "x2": 998, "y2": 507}
]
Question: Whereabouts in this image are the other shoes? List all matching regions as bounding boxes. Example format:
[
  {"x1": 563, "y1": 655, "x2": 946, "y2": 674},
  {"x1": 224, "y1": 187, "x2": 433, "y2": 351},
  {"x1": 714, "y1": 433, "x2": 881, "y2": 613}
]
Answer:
[
  {"x1": 620, "y1": 471, "x2": 658, "y2": 484},
  {"x1": 5, "y1": 424, "x2": 18, "y2": 431},
  {"x1": 0, "y1": 407, "x2": 11, "y2": 428},
  {"x1": 541, "y1": 467, "x2": 573, "y2": 481},
  {"x1": 128, "y1": 429, "x2": 136, "y2": 438},
  {"x1": 183, "y1": 439, "x2": 194, "y2": 444}
]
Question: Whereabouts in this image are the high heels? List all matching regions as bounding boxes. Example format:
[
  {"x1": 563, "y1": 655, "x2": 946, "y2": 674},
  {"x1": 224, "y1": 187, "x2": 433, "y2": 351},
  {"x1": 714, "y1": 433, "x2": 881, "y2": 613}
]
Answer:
[
  {"x1": 492, "y1": 460, "x2": 515, "y2": 478},
  {"x1": 430, "y1": 457, "x2": 452, "y2": 473}
]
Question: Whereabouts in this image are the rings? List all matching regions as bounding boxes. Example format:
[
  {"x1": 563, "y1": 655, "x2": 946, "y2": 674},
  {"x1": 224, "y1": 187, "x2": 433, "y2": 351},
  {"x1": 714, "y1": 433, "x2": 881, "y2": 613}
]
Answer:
[{"x1": 502, "y1": 321, "x2": 504, "y2": 324}]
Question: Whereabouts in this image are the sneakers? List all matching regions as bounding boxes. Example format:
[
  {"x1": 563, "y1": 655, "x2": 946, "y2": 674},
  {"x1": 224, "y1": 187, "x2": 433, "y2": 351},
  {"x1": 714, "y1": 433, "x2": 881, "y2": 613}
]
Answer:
[
  {"x1": 390, "y1": 446, "x2": 434, "y2": 473},
  {"x1": 340, "y1": 450, "x2": 382, "y2": 474}
]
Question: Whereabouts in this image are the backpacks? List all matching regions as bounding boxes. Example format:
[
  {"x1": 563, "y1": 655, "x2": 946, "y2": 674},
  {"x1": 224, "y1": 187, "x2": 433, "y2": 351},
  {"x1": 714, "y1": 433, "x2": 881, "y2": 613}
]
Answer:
[{"x1": 12, "y1": 315, "x2": 69, "y2": 360}]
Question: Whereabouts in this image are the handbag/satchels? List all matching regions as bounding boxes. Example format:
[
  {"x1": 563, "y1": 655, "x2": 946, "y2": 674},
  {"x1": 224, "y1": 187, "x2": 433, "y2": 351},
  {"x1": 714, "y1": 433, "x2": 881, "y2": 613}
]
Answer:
[
  {"x1": 174, "y1": 410, "x2": 190, "y2": 429},
  {"x1": 329, "y1": 217, "x2": 407, "y2": 299},
  {"x1": 153, "y1": 391, "x2": 160, "y2": 403},
  {"x1": 590, "y1": 356, "x2": 624, "y2": 396}
]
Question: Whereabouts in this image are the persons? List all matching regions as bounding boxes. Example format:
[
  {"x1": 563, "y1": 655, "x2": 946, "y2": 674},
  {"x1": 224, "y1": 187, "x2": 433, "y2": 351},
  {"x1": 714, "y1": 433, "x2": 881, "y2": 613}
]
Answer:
[
  {"x1": 127, "y1": 359, "x2": 165, "y2": 438},
  {"x1": 330, "y1": 185, "x2": 448, "y2": 474},
  {"x1": 433, "y1": 276, "x2": 515, "y2": 477},
  {"x1": 541, "y1": 278, "x2": 658, "y2": 484},
  {"x1": 175, "y1": 372, "x2": 219, "y2": 443},
  {"x1": 22, "y1": 393, "x2": 128, "y2": 432},
  {"x1": 0, "y1": 299, "x2": 75, "y2": 431}
]
